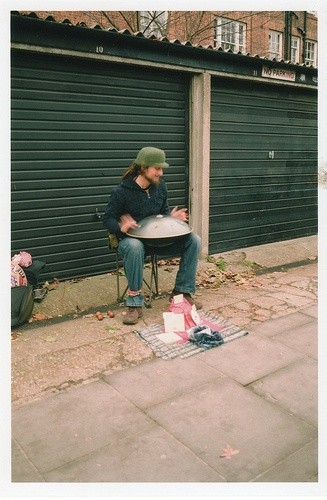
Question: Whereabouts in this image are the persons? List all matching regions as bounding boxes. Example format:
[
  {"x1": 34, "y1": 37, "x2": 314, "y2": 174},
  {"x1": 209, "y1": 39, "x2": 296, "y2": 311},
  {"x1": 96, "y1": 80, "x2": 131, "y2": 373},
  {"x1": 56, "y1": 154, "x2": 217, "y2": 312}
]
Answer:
[{"x1": 103, "y1": 147, "x2": 202, "y2": 324}]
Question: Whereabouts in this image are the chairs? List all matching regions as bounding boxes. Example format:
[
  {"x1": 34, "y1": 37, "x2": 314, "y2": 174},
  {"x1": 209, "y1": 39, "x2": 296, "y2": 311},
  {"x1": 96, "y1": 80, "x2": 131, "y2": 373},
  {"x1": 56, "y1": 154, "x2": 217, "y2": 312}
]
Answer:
[{"x1": 105, "y1": 228, "x2": 167, "y2": 309}]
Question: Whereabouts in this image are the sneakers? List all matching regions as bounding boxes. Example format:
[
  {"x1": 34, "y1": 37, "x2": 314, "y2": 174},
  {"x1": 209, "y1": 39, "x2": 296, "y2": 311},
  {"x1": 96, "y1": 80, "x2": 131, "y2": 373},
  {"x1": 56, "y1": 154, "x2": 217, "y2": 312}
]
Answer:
[
  {"x1": 123, "y1": 307, "x2": 143, "y2": 324},
  {"x1": 169, "y1": 290, "x2": 203, "y2": 310}
]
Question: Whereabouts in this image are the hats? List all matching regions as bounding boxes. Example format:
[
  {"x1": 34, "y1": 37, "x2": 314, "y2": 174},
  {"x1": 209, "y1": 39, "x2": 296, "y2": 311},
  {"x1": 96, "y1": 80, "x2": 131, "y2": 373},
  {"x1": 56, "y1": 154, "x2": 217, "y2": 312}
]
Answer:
[{"x1": 134, "y1": 146, "x2": 170, "y2": 168}]
